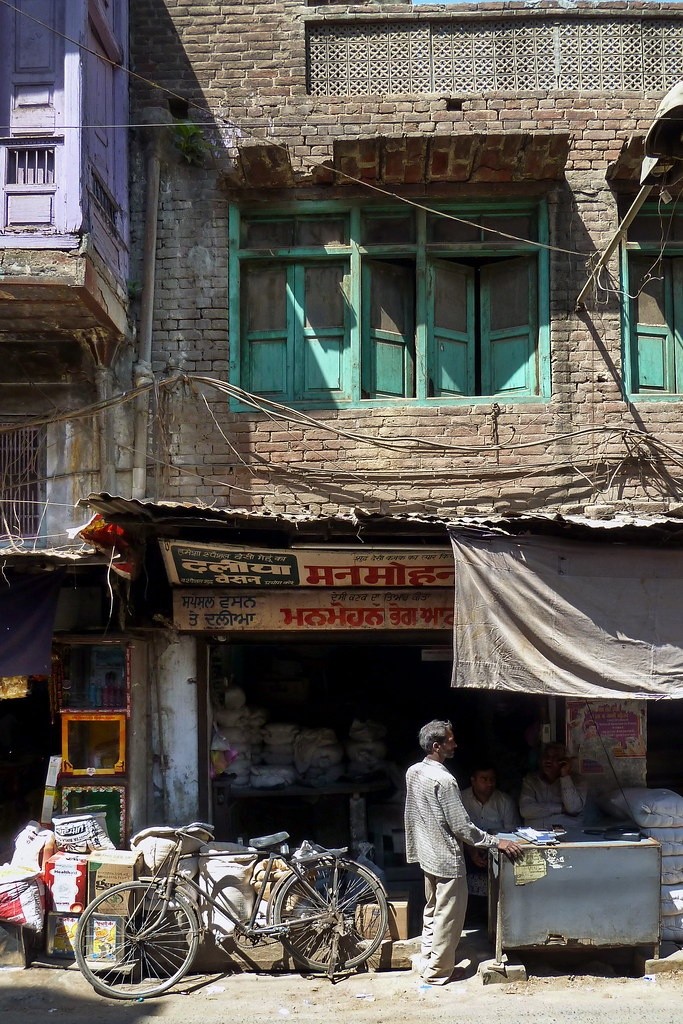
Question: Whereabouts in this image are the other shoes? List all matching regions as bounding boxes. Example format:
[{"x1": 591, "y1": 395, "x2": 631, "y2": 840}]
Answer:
[{"x1": 446, "y1": 967, "x2": 467, "y2": 982}]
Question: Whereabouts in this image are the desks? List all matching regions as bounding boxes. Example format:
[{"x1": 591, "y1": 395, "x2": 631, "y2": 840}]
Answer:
[{"x1": 486, "y1": 830, "x2": 664, "y2": 963}]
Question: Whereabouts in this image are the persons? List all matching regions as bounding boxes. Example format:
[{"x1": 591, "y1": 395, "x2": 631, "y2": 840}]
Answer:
[
  {"x1": 520, "y1": 738, "x2": 588, "y2": 831},
  {"x1": 404, "y1": 721, "x2": 524, "y2": 985},
  {"x1": 458, "y1": 759, "x2": 519, "y2": 896}
]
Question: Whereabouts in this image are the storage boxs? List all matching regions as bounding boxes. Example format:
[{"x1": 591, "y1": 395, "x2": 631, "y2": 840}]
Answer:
[
  {"x1": 355, "y1": 890, "x2": 411, "y2": 940},
  {"x1": 44, "y1": 850, "x2": 89, "y2": 913},
  {"x1": 87, "y1": 848, "x2": 144, "y2": 916},
  {"x1": 86, "y1": 915, "x2": 125, "y2": 962},
  {"x1": 45, "y1": 911, "x2": 85, "y2": 960}
]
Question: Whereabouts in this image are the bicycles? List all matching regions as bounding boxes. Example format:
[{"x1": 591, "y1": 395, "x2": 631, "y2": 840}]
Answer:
[{"x1": 74, "y1": 821, "x2": 390, "y2": 1001}]
